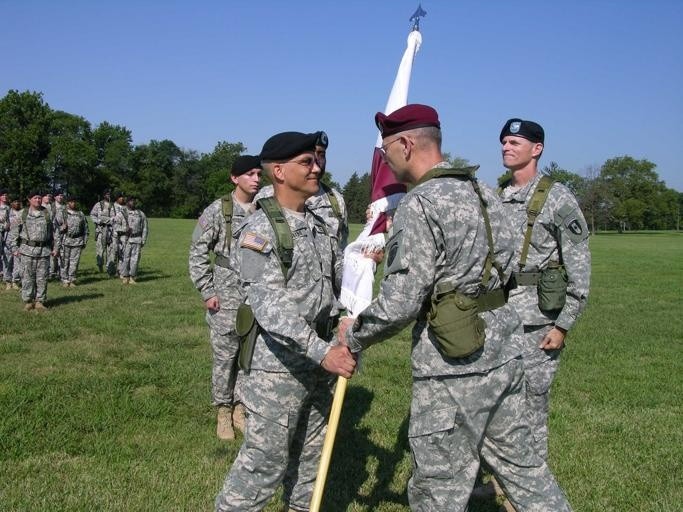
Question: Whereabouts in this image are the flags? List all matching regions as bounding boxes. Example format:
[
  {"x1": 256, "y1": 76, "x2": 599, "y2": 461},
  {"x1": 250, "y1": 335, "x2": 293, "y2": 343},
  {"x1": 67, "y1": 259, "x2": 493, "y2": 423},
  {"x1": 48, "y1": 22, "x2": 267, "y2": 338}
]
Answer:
[{"x1": 340, "y1": 27, "x2": 414, "y2": 326}]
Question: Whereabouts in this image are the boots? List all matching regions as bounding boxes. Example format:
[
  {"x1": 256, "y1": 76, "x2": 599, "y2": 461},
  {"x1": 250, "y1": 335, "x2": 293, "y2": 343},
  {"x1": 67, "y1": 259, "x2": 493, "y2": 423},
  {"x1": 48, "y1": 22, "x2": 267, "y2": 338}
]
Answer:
[
  {"x1": 232, "y1": 404, "x2": 246, "y2": 432},
  {"x1": 217, "y1": 407, "x2": 235, "y2": 442}
]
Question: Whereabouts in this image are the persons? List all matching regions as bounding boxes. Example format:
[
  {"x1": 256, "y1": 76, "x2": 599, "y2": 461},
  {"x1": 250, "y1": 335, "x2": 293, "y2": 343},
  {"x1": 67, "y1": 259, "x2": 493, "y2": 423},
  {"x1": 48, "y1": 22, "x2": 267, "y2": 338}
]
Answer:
[
  {"x1": 474, "y1": 117, "x2": 593, "y2": 512},
  {"x1": 214, "y1": 131, "x2": 360, "y2": 511},
  {"x1": 187, "y1": 156, "x2": 264, "y2": 443},
  {"x1": 299, "y1": 130, "x2": 348, "y2": 259},
  {"x1": 340, "y1": 103, "x2": 577, "y2": 510},
  {"x1": 0, "y1": 182, "x2": 151, "y2": 313}
]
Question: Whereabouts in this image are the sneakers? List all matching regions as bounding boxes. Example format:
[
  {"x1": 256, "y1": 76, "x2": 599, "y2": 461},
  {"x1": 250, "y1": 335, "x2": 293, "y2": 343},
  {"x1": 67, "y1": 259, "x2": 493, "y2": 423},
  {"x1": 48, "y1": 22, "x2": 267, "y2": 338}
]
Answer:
[
  {"x1": 123, "y1": 277, "x2": 136, "y2": 285},
  {"x1": 62, "y1": 283, "x2": 76, "y2": 288},
  {"x1": 23, "y1": 302, "x2": 47, "y2": 311},
  {"x1": 4, "y1": 280, "x2": 20, "y2": 290}
]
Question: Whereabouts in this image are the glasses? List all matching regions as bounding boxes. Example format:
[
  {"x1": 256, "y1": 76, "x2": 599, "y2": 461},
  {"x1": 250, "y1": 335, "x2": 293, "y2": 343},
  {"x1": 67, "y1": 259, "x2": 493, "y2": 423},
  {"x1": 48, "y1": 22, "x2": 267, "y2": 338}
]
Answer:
[
  {"x1": 280, "y1": 157, "x2": 314, "y2": 166},
  {"x1": 378, "y1": 138, "x2": 402, "y2": 158}
]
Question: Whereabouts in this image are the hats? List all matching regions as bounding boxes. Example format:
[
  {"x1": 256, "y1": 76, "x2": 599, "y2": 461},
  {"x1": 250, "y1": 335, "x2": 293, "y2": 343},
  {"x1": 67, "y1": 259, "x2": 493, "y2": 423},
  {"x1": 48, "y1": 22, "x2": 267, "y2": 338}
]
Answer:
[
  {"x1": 231, "y1": 155, "x2": 263, "y2": 175},
  {"x1": 315, "y1": 131, "x2": 328, "y2": 150},
  {"x1": 102, "y1": 187, "x2": 137, "y2": 203},
  {"x1": 375, "y1": 104, "x2": 441, "y2": 138},
  {"x1": 0, "y1": 187, "x2": 77, "y2": 202},
  {"x1": 500, "y1": 118, "x2": 544, "y2": 144},
  {"x1": 260, "y1": 132, "x2": 318, "y2": 160}
]
